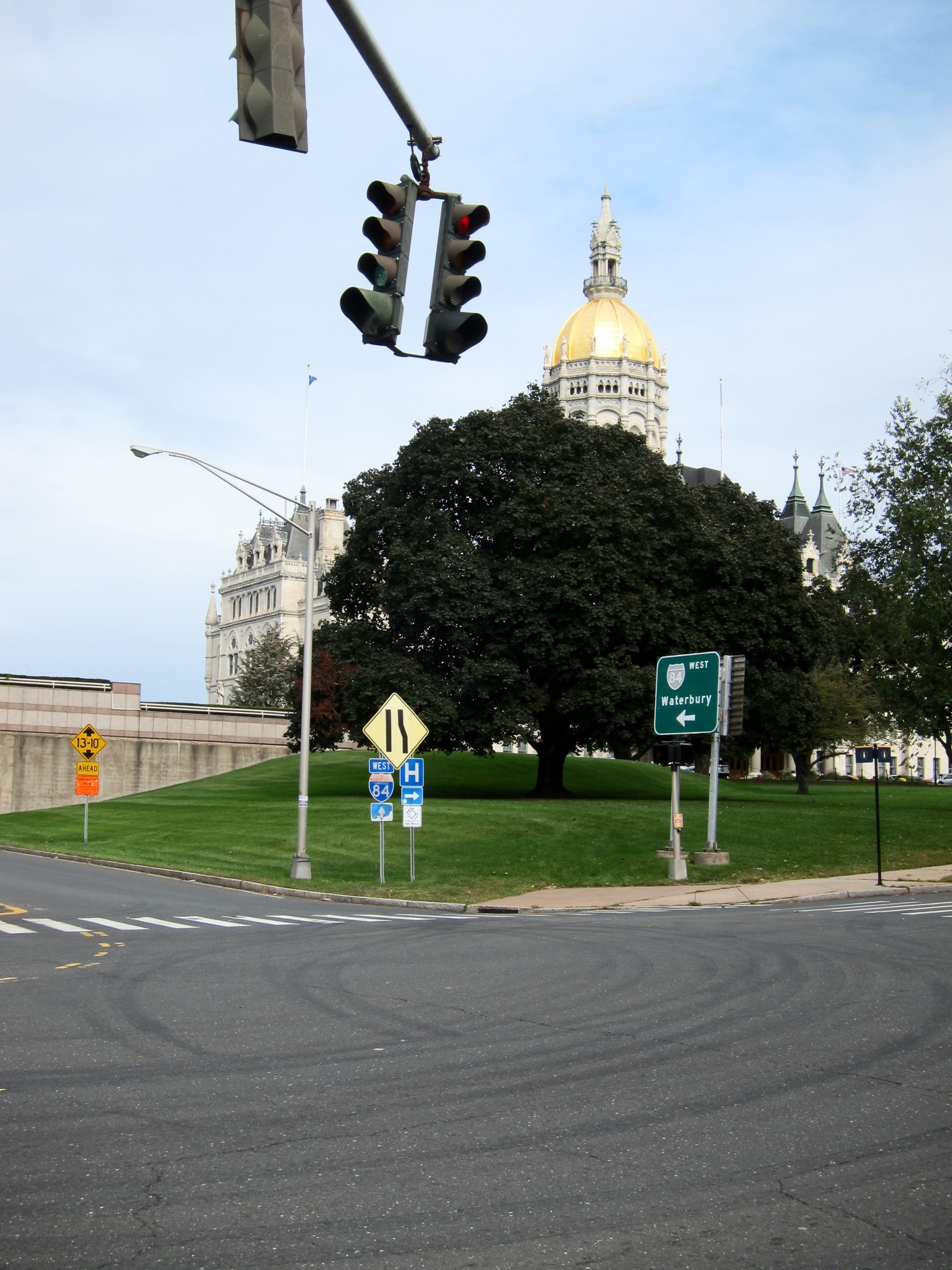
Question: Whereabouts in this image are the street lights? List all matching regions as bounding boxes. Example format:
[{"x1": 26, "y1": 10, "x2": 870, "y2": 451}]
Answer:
[{"x1": 131, "y1": 444, "x2": 317, "y2": 880}]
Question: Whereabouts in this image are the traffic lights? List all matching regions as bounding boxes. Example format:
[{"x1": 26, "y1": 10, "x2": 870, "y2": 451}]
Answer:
[
  {"x1": 428, "y1": 203, "x2": 490, "y2": 360},
  {"x1": 340, "y1": 182, "x2": 407, "y2": 335}
]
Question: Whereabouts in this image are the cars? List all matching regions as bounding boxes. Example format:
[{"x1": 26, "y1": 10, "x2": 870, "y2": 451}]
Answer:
[
  {"x1": 675, "y1": 758, "x2": 731, "y2": 779},
  {"x1": 929, "y1": 773, "x2": 952, "y2": 785}
]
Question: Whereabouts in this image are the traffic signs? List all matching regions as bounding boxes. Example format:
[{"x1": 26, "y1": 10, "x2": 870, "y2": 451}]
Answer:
[{"x1": 655, "y1": 651, "x2": 718, "y2": 734}]
